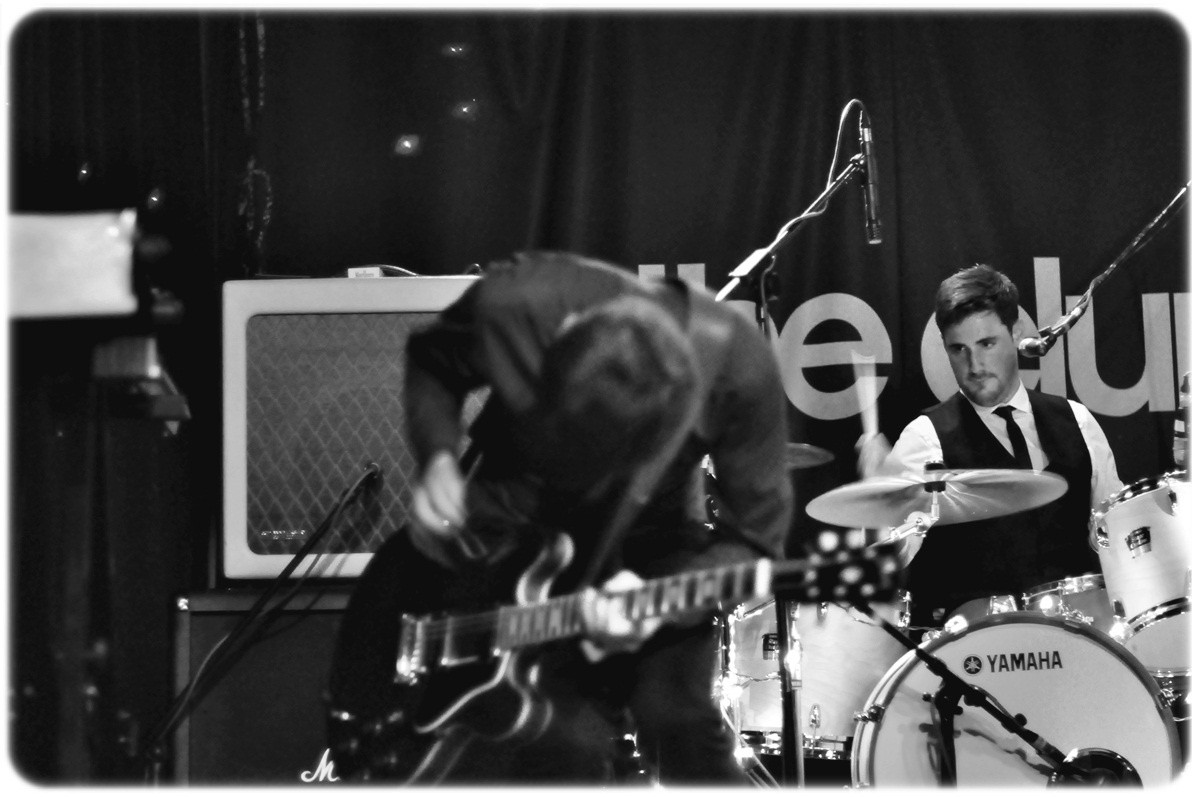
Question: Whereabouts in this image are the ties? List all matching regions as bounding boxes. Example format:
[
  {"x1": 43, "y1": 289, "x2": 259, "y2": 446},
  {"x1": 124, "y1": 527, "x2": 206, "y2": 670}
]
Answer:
[{"x1": 993, "y1": 405, "x2": 1032, "y2": 469}]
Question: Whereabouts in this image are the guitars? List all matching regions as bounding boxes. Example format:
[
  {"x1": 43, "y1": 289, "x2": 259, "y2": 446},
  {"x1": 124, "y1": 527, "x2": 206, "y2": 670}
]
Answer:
[{"x1": 328, "y1": 514, "x2": 906, "y2": 787}]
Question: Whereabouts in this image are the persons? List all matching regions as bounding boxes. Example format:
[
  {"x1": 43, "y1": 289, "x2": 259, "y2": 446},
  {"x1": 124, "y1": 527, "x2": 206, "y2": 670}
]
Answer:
[
  {"x1": 869, "y1": 265, "x2": 1134, "y2": 644},
  {"x1": 399, "y1": 250, "x2": 798, "y2": 784}
]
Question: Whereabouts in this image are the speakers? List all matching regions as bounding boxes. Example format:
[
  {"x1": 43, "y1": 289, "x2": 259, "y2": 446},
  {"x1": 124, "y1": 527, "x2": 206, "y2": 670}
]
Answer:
[
  {"x1": 174, "y1": 590, "x2": 359, "y2": 789},
  {"x1": 221, "y1": 275, "x2": 490, "y2": 579}
]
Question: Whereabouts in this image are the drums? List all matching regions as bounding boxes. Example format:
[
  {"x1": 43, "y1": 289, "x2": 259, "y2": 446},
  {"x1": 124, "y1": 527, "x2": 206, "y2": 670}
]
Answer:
[
  {"x1": 1088, "y1": 469, "x2": 1187, "y2": 678},
  {"x1": 712, "y1": 588, "x2": 911, "y2": 763},
  {"x1": 1020, "y1": 574, "x2": 1115, "y2": 636},
  {"x1": 851, "y1": 612, "x2": 1181, "y2": 785}
]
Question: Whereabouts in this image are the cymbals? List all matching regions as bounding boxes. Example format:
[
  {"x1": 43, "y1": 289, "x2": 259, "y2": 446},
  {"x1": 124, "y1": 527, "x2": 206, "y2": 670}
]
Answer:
[
  {"x1": 806, "y1": 468, "x2": 1069, "y2": 528},
  {"x1": 704, "y1": 443, "x2": 834, "y2": 478}
]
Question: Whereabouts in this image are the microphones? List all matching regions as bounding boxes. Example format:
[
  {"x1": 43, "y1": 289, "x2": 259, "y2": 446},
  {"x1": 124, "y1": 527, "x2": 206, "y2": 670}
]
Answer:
[
  {"x1": 363, "y1": 459, "x2": 385, "y2": 498},
  {"x1": 859, "y1": 110, "x2": 882, "y2": 244},
  {"x1": 1018, "y1": 338, "x2": 1046, "y2": 357},
  {"x1": 1021, "y1": 729, "x2": 1120, "y2": 787}
]
